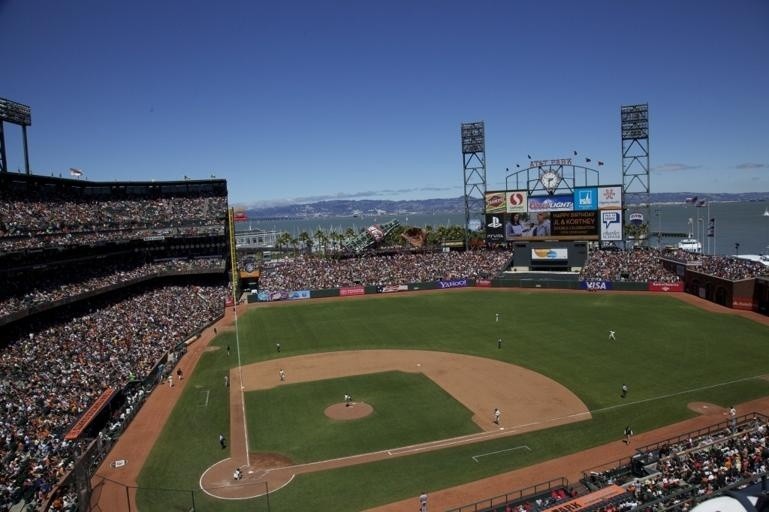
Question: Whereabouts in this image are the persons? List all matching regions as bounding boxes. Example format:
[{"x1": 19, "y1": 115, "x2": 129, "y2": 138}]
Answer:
[
  {"x1": 348, "y1": 393, "x2": 353, "y2": 403},
  {"x1": 279, "y1": 368, "x2": 285, "y2": 382},
  {"x1": 495, "y1": 312, "x2": 499, "y2": 322},
  {"x1": 729, "y1": 406, "x2": 736, "y2": 415},
  {"x1": 234, "y1": 244, "x2": 768, "y2": 294},
  {"x1": 276, "y1": 343, "x2": 281, "y2": 353},
  {"x1": 494, "y1": 408, "x2": 501, "y2": 424},
  {"x1": 532, "y1": 212, "x2": 551, "y2": 238},
  {"x1": 1, "y1": 180, "x2": 232, "y2": 511},
  {"x1": 344, "y1": 393, "x2": 348, "y2": 405},
  {"x1": 608, "y1": 329, "x2": 616, "y2": 343},
  {"x1": 505, "y1": 213, "x2": 525, "y2": 237},
  {"x1": 493, "y1": 411, "x2": 768, "y2": 511},
  {"x1": 496, "y1": 336, "x2": 502, "y2": 349},
  {"x1": 234, "y1": 468, "x2": 240, "y2": 481},
  {"x1": 620, "y1": 383, "x2": 628, "y2": 398},
  {"x1": 418, "y1": 490, "x2": 428, "y2": 512}
]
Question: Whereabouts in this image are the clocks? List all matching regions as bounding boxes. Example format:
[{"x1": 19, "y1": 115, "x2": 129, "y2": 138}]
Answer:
[{"x1": 540, "y1": 170, "x2": 560, "y2": 189}]
